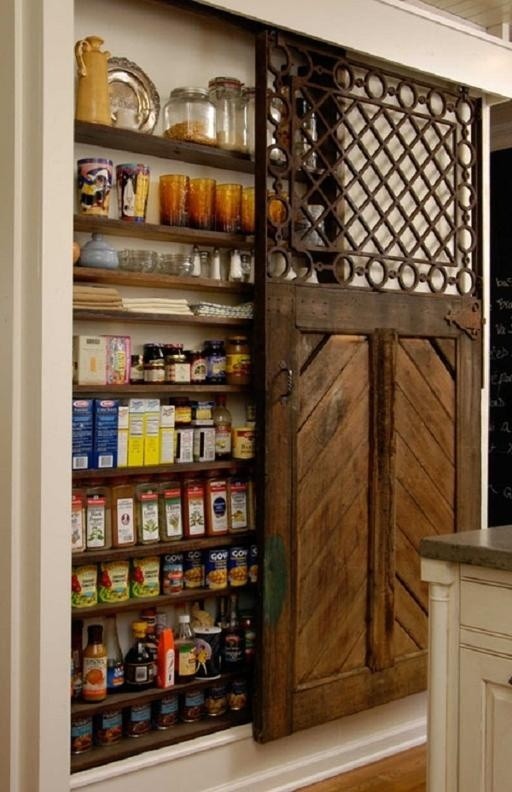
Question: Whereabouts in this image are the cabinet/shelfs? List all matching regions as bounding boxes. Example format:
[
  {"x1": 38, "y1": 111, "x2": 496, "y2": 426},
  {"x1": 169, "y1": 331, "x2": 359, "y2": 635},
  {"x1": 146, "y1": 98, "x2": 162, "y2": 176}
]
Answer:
[
  {"x1": 419, "y1": 524, "x2": 512, "y2": 792},
  {"x1": 70, "y1": 0, "x2": 343, "y2": 775}
]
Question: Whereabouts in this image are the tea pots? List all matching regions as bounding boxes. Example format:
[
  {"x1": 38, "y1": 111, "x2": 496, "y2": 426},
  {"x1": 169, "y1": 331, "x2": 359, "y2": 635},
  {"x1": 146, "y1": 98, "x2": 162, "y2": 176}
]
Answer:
[{"x1": 75, "y1": 34, "x2": 113, "y2": 128}]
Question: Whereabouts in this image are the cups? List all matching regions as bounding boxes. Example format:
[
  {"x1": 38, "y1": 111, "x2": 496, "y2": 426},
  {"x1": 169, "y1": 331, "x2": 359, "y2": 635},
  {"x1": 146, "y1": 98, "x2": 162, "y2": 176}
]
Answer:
[{"x1": 77, "y1": 158, "x2": 327, "y2": 245}]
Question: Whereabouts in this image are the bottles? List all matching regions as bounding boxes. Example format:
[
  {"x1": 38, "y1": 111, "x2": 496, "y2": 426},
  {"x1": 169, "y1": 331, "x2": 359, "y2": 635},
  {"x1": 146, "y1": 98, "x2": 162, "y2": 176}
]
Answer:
[
  {"x1": 165, "y1": 78, "x2": 317, "y2": 171},
  {"x1": 214, "y1": 394, "x2": 236, "y2": 459},
  {"x1": 73, "y1": 232, "x2": 322, "y2": 285},
  {"x1": 72, "y1": 595, "x2": 263, "y2": 699},
  {"x1": 71, "y1": 472, "x2": 263, "y2": 555}
]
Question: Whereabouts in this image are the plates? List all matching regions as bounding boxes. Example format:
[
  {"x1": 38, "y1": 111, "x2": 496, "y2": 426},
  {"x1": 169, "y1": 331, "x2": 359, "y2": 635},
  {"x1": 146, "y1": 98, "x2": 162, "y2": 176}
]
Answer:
[{"x1": 108, "y1": 56, "x2": 160, "y2": 138}]
